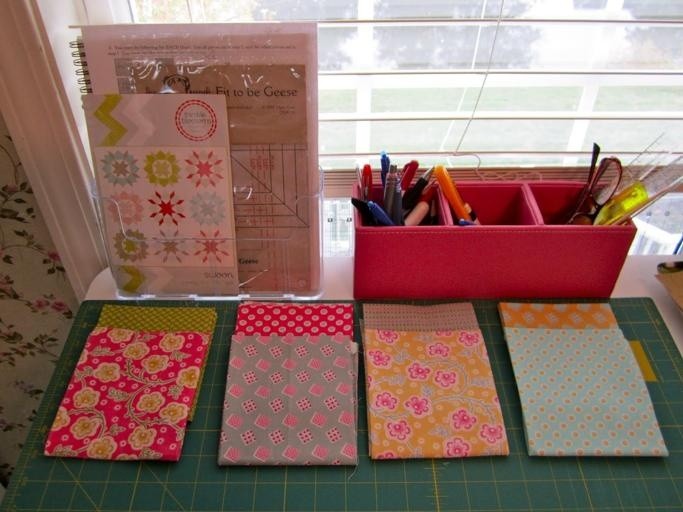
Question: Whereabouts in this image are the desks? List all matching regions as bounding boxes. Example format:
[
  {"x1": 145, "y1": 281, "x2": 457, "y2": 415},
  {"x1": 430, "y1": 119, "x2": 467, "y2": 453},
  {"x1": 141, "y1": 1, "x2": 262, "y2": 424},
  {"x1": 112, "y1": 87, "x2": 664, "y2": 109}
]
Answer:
[{"x1": 1, "y1": 254, "x2": 682, "y2": 508}]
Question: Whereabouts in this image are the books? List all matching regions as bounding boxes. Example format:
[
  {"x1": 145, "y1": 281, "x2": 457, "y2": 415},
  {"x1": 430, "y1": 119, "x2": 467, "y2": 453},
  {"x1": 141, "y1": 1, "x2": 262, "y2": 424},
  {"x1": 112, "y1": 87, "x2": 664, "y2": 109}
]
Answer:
[{"x1": 70, "y1": 21, "x2": 327, "y2": 300}]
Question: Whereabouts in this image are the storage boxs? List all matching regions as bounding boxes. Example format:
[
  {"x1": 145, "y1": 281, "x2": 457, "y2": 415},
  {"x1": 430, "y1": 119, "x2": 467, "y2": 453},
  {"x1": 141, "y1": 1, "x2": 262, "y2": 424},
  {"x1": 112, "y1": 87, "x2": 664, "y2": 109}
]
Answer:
[{"x1": 352, "y1": 180, "x2": 637, "y2": 301}]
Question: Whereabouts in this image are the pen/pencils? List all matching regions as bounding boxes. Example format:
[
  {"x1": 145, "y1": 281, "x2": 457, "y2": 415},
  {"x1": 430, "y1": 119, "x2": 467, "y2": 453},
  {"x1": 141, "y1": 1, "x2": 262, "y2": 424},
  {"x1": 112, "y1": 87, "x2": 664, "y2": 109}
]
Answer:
[{"x1": 351, "y1": 151, "x2": 480, "y2": 226}]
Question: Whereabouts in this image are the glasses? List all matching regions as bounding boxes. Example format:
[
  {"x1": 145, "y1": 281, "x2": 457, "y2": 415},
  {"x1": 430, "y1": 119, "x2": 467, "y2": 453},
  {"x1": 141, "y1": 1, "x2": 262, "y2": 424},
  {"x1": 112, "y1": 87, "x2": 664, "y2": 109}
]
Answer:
[{"x1": 565, "y1": 143, "x2": 622, "y2": 225}]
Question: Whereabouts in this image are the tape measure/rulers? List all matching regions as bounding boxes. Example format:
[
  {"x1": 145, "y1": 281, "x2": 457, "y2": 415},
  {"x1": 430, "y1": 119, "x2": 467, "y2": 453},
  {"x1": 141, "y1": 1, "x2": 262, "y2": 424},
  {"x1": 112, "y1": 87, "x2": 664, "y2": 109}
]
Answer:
[
  {"x1": 613, "y1": 128, "x2": 672, "y2": 195},
  {"x1": 612, "y1": 157, "x2": 683, "y2": 225}
]
